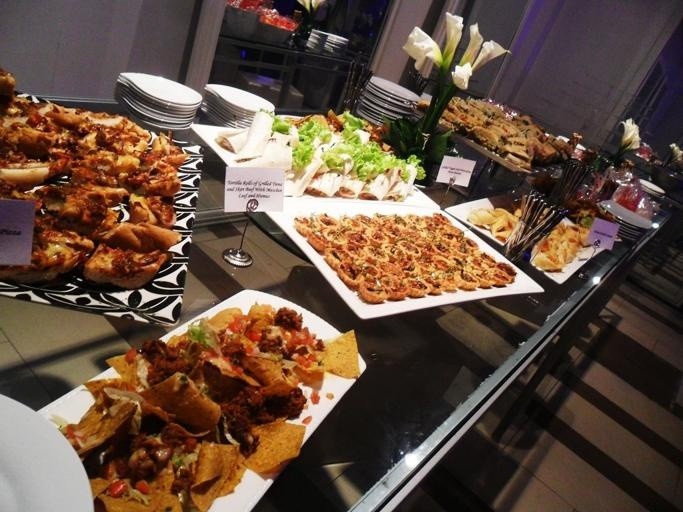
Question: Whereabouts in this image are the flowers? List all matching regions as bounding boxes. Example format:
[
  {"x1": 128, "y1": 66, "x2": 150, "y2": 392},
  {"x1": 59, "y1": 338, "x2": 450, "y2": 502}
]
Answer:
[
  {"x1": 297, "y1": 0, "x2": 326, "y2": 24},
  {"x1": 407, "y1": 15, "x2": 512, "y2": 132}
]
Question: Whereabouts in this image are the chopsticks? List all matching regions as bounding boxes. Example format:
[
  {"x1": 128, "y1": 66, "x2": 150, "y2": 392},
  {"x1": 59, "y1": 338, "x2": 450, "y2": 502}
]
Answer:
[
  {"x1": 504, "y1": 189, "x2": 569, "y2": 265},
  {"x1": 554, "y1": 158, "x2": 596, "y2": 201}
]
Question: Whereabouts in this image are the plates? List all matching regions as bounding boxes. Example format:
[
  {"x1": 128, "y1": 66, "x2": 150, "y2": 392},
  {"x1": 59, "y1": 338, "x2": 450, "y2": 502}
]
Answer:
[
  {"x1": 30, "y1": 289, "x2": 367, "y2": 512},
  {"x1": 267, "y1": 193, "x2": 545, "y2": 320},
  {"x1": 200, "y1": 82, "x2": 275, "y2": 128},
  {"x1": 356, "y1": 75, "x2": 421, "y2": 129},
  {"x1": 597, "y1": 199, "x2": 653, "y2": 241},
  {"x1": 445, "y1": 181, "x2": 608, "y2": 286},
  {"x1": 308, "y1": 28, "x2": 349, "y2": 57},
  {"x1": 0, "y1": 393, "x2": 93, "y2": 512},
  {"x1": 2, "y1": 89, "x2": 205, "y2": 328},
  {"x1": 113, "y1": 71, "x2": 202, "y2": 130},
  {"x1": 636, "y1": 177, "x2": 668, "y2": 200},
  {"x1": 189, "y1": 122, "x2": 440, "y2": 208}
]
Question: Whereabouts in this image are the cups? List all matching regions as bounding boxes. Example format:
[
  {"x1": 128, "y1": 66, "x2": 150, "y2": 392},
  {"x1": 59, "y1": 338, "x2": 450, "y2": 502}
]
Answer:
[{"x1": 499, "y1": 217, "x2": 551, "y2": 273}]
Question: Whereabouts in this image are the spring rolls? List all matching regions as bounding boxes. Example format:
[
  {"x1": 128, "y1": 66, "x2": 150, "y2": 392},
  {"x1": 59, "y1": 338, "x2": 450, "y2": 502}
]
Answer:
[{"x1": 216, "y1": 108, "x2": 426, "y2": 201}]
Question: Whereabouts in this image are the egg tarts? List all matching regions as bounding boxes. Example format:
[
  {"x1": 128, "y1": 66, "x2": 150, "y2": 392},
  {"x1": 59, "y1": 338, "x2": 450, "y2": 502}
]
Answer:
[{"x1": 293, "y1": 214, "x2": 517, "y2": 303}]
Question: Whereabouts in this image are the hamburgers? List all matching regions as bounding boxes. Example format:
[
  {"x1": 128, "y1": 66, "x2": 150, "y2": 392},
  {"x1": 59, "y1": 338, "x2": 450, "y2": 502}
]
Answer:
[{"x1": 419, "y1": 98, "x2": 572, "y2": 172}]
